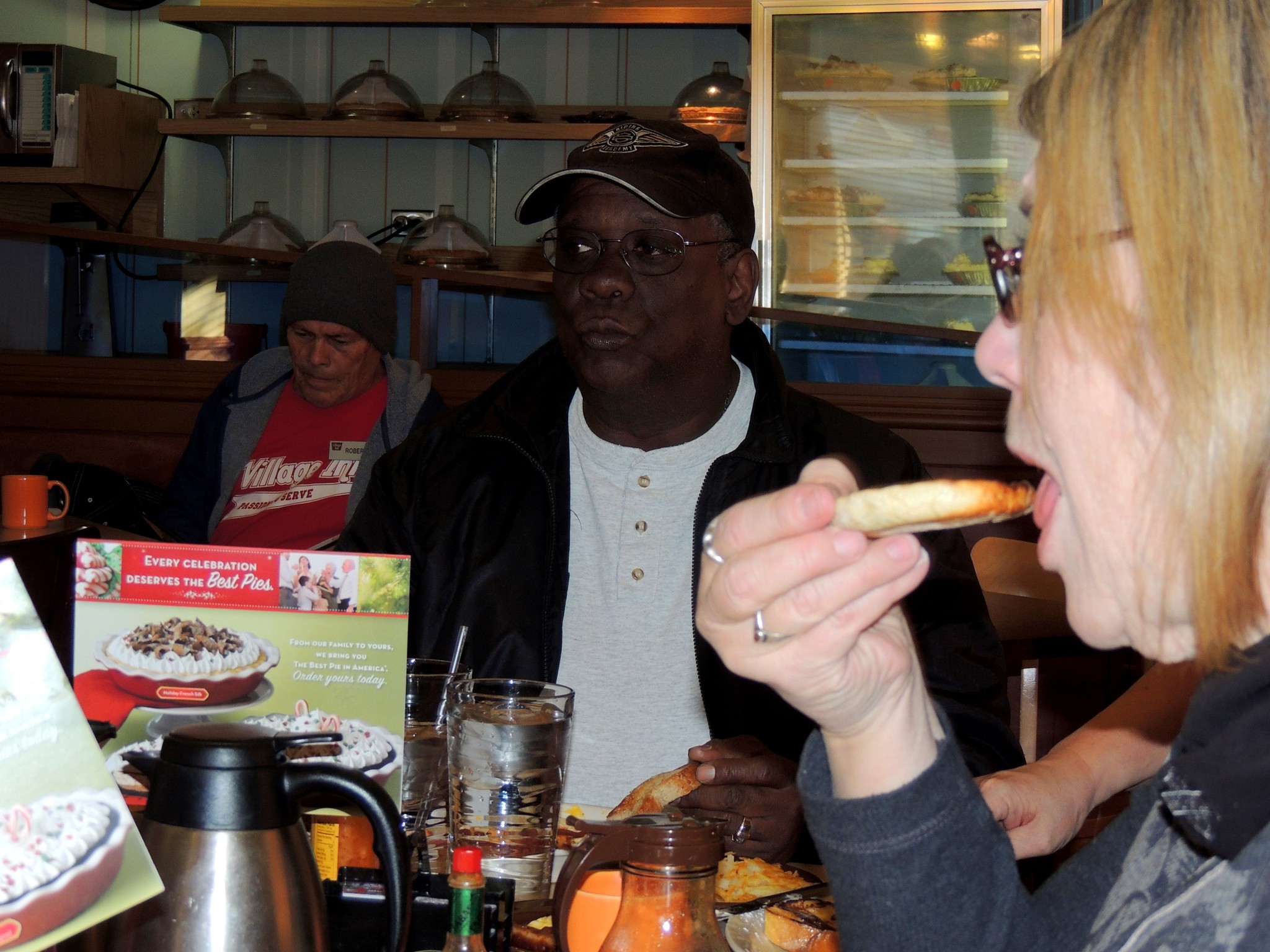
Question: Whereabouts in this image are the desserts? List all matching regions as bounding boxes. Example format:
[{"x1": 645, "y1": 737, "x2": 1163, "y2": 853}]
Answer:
[{"x1": 0, "y1": 616, "x2": 395, "y2": 905}]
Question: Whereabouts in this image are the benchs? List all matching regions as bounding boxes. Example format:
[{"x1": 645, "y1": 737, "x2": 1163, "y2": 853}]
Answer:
[{"x1": 0, "y1": 343, "x2": 1145, "y2": 852}]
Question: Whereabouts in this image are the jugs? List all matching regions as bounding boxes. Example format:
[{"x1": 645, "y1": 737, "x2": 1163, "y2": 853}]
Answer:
[
  {"x1": 83, "y1": 722, "x2": 412, "y2": 952},
  {"x1": 552, "y1": 809, "x2": 734, "y2": 951}
]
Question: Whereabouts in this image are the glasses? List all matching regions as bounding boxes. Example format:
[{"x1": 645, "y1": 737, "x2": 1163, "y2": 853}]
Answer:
[
  {"x1": 983, "y1": 225, "x2": 1135, "y2": 329},
  {"x1": 536, "y1": 226, "x2": 744, "y2": 276}
]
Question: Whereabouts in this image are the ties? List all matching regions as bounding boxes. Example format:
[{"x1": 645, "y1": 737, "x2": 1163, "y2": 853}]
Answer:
[{"x1": 336, "y1": 574, "x2": 348, "y2": 604}]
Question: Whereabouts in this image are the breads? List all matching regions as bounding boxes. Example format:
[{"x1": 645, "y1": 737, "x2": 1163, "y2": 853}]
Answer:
[
  {"x1": 764, "y1": 899, "x2": 840, "y2": 952},
  {"x1": 606, "y1": 760, "x2": 702, "y2": 820},
  {"x1": 827, "y1": 478, "x2": 1036, "y2": 541}
]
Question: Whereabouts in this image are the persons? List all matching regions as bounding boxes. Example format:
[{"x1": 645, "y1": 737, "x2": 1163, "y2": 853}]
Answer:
[
  {"x1": 959, "y1": 612, "x2": 1237, "y2": 860},
  {"x1": 104, "y1": 235, "x2": 456, "y2": 573},
  {"x1": 279, "y1": 552, "x2": 357, "y2": 613},
  {"x1": 696, "y1": 1, "x2": 1266, "y2": 952},
  {"x1": 328, "y1": 113, "x2": 1041, "y2": 910}
]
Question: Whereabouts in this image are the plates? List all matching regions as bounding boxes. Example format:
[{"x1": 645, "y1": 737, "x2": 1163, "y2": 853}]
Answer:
[
  {"x1": 714, "y1": 854, "x2": 824, "y2": 916},
  {"x1": 93, "y1": 628, "x2": 279, "y2": 703},
  {"x1": 788, "y1": 200, "x2": 883, "y2": 217},
  {"x1": 909, "y1": 75, "x2": 1008, "y2": 92},
  {"x1": 941, "y1": 270, "x2": 994, "y2": 286},
  {"x1": 807, "y1": 273, "x2": 900, "y2": 285},
  {"x1": 0, "y1": 790, "x2": 132, "y2": 951},
  {"x1": 948, "y1": 201, "x2": 1007, "y2": 218},
  {"x1": 724, "y1": 893, "x2": 839, "y2": 952},
  {"x1": 251, "y1": 713, "x2": 402, "y2": 788},
  {"x1": 791, "y1": 74, "x2": 893, "y2": 91},
  {"x1": 817, "y1": 144, "x2": 892, "y2": 160}
]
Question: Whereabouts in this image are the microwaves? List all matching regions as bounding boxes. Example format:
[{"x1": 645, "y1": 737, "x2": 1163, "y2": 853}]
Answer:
[{"x1": 0, "y1": 41, "x2": 118, "y2": 167}]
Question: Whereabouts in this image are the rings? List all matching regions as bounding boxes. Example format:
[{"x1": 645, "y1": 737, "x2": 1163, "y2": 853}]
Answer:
[
  {"x1": 702, "y1": 510, "x2": 730, "y2": 567},
  {"x1": 752, "y1": 611, "x2": 791, "y2": 645}
]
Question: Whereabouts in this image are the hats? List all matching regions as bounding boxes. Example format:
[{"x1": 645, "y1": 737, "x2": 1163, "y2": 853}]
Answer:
[
  {"x1": 280, "y1": 240, "x2": 399, "y2": 356},
  {"x1": 514, "y1": 120, "x2": 755, "y2": 249}
]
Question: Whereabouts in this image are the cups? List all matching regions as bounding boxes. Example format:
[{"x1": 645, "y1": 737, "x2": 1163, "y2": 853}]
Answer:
[
  {"x1": 1, "y1": 476, "x2": 70, "y2": 529},
  {"x1": 400, "y1": 658, "x2": 474, "y2": 877},
  {"x1": 444, "y1": 676, "x2": 575, "y2": 913}
]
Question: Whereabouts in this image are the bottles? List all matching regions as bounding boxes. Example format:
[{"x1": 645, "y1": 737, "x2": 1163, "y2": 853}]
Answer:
[{"x1": 438, "y1": 845, "x2": 489, "y2": 951}]
